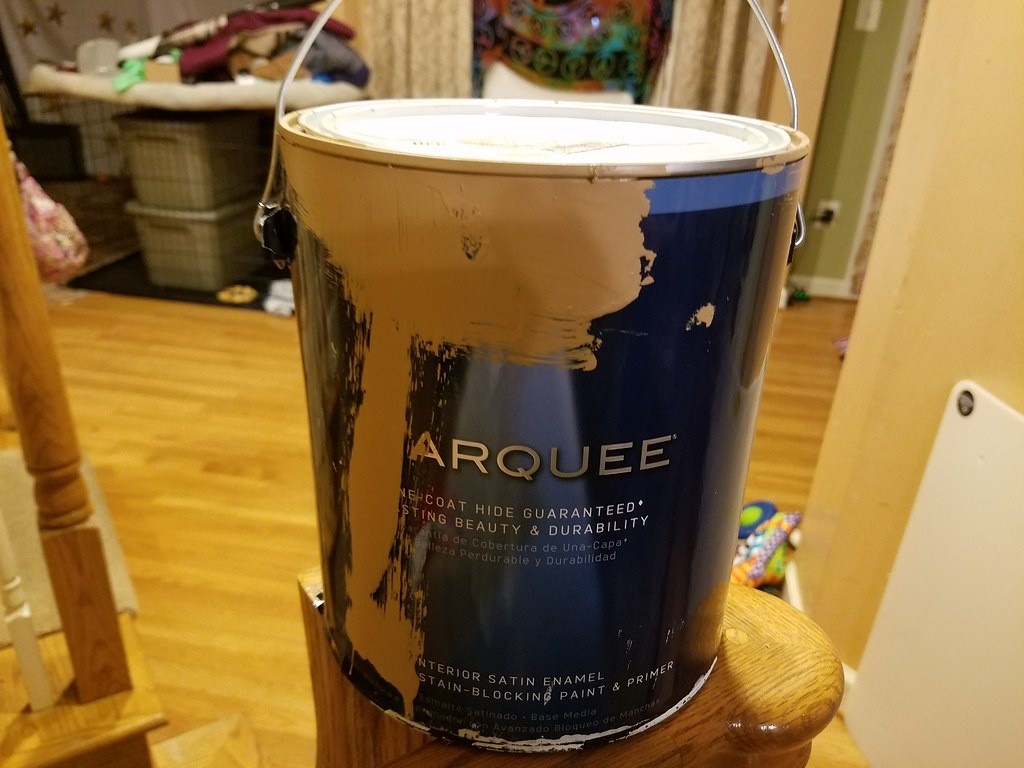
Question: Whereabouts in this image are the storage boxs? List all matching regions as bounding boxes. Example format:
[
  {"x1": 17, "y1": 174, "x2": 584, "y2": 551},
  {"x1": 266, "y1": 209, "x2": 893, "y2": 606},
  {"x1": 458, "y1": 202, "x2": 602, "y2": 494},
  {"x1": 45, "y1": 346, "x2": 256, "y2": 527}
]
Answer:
[
  {"x1": 112, "y1": 106, "x2": 265, "y2": 214},
  {"x1": 123, "y1": 192, "x2": 264, "y2": 291}
]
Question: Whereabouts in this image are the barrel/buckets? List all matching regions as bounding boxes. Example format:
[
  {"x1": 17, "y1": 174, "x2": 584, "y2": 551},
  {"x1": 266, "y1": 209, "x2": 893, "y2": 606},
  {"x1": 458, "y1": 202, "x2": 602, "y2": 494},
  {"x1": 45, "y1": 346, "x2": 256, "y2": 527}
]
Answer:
[{"x1": 251, "y1": 2, "x2": 814, "y2": 756}]
